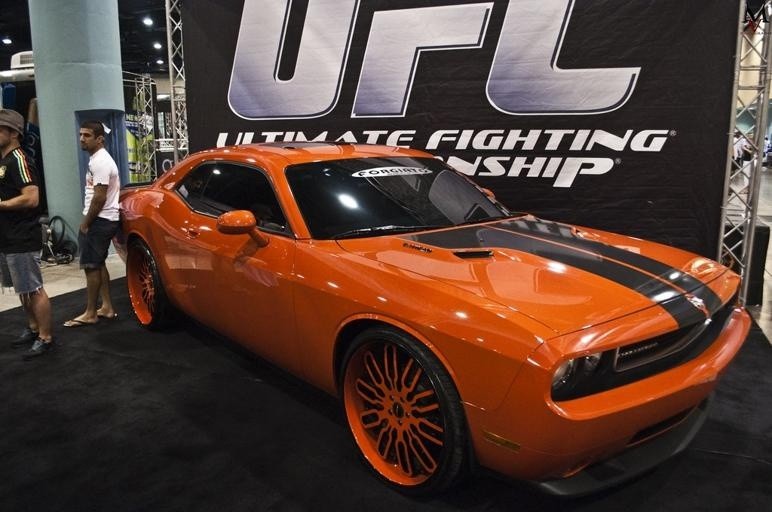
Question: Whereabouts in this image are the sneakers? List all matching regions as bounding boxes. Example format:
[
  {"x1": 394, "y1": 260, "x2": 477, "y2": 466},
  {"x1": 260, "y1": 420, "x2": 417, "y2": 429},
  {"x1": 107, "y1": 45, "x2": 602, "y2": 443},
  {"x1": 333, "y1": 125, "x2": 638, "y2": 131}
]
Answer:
[
  {"x1": 21, "y1": 336, "x2": 50, "y2": 355},
  {"x1": 12, "y1": 328, "x2": 39, "y2": 345}
]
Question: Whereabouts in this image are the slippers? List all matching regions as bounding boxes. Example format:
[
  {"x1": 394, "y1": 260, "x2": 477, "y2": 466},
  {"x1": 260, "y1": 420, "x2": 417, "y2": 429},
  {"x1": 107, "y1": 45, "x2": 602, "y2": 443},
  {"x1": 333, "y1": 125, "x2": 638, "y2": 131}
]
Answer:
[{"x1": 63, "y1": 318, "x2": 92, "y2": 328}]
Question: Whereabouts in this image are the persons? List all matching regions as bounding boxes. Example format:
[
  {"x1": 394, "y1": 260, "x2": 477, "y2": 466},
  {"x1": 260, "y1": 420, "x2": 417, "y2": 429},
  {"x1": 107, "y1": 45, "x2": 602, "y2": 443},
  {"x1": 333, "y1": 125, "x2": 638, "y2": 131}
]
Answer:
[
  {"x1": 732, "y1": 130, "x2": 772, "y2": 174},
  {"x1": 63, "y1": 119, "x2": 120, "y2": 328},
  {"x1": 0, "y1": 107, "x2": 53, "y2": 360}
]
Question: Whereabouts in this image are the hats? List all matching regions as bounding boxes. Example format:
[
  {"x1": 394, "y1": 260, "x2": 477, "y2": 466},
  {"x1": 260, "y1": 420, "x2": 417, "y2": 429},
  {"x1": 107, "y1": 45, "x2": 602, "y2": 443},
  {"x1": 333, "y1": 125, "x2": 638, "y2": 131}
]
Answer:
[{"x1": 0, "y1": 108, "x2": 26, "y2": 136}]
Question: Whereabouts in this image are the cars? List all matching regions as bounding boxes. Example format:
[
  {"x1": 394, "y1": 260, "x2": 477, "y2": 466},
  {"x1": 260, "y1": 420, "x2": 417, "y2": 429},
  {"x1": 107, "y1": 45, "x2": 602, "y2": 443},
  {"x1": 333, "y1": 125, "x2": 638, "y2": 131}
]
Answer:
[{"x1": 110, "y1": 141, "x2": 753, "y2": 497}]
